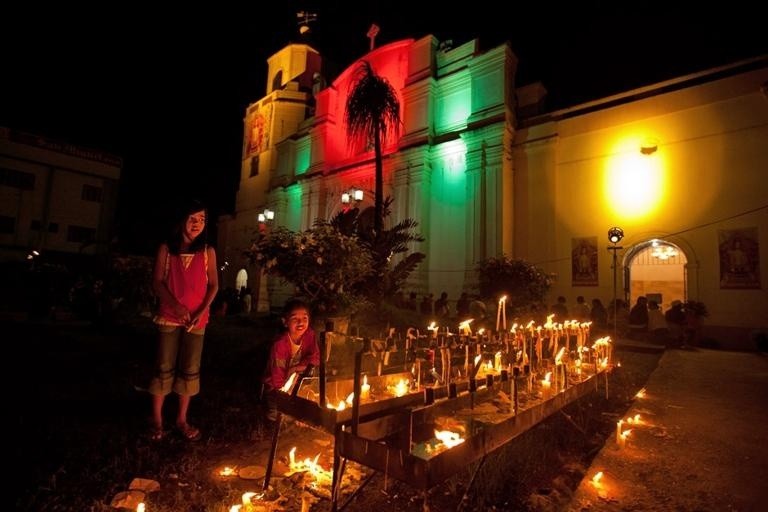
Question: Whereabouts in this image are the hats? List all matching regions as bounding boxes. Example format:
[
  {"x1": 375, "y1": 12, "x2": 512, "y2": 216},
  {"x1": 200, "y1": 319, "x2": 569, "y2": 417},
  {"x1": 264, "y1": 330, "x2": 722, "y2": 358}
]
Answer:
[{"x1": 671, "y1": 300, "x2": 683, "y2": 307}]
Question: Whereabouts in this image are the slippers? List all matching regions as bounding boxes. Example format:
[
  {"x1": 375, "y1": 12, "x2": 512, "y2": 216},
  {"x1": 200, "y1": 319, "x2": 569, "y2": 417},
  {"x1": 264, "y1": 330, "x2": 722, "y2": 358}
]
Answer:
[
  {"x1": 151, "y1": 428, "x2": 163, "y2": 442},
  {"x1": 184, "y1": 426, "x2": 202, "y2": 441}
]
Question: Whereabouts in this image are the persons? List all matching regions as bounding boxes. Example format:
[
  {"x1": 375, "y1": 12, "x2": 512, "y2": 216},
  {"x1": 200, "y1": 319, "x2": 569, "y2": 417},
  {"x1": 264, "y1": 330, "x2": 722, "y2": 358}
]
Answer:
[
  {"x1": 392, "y1": 290, "x2": 487, "y2": 321},
  {"x1": 146, "y1": 200, "x2": 220, "y2": 442},
  {"x1": 577, "y1": 245, "x2": 592, "y2": 273},
  {"x1": 210, "y1": 286, "x2": 253, "y2": 315},
  {"x1": 242, "y1": 298, "x2": 332, "y2": 444},
  {"x1": 551, "y1": 295, "x2": 697, "y2": 350},
  {"x1": 728, "y1": 237, "x2": 750, "y2": 273}
]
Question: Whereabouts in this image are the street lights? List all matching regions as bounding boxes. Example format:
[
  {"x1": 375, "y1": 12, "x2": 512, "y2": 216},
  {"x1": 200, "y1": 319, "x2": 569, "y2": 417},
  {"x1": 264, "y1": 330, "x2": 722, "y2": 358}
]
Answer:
[{"x1": 606, "y1": 226, "x2": 624, "y2": 350}]
